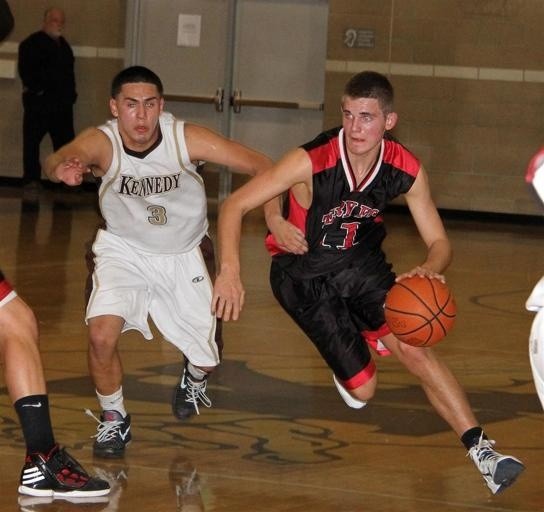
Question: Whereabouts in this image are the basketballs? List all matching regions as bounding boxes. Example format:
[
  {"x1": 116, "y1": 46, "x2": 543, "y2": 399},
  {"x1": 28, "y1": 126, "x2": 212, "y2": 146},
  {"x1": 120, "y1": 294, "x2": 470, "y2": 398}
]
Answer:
[{"x1": 384, "y1": 275, "x2": 457, "y2": 347}]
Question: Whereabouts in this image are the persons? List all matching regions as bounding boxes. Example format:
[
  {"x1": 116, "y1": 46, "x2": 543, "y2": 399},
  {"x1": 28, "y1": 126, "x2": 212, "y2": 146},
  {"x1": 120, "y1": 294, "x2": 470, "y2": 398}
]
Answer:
[
  {"x1": 525, "y1": 149, "x2": 544, "y2": 411},
  {"x1": 0, "y1": 266, "x2": 112, "y2": 498},
  {"x1": 17, "y1": 8, "x2": 79, "y2": 207},
  {"x1": 211, "y1": 71, "x2": 526, "y2": 496},
  {"x1": 41, "y1": 66, "x2": 309, "y2": 459}
]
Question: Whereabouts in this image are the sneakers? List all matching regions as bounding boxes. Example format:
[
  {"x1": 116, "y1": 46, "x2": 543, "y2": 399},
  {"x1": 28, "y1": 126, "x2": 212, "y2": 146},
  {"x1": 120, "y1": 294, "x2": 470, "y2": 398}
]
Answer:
[
  {"x1": 17, "y1": 442, "x2": 112, "y2": 498},
  {"x1": 17, "y1": 497, "x2": 110, "y2": 512},
  {"x1": 172, "y1": 352, "x2": 211, "y2": 420},
  {"x1": 464, "y1": 427, "x2": 526, "y2": 494},
  {"x1": 168, "y1": 456, "x2": 204, "y2": 512},
  {"x1": 94, "y1": 409, "x2": 131, "y2": 459}
]
